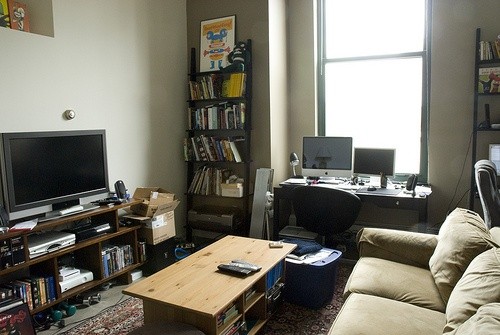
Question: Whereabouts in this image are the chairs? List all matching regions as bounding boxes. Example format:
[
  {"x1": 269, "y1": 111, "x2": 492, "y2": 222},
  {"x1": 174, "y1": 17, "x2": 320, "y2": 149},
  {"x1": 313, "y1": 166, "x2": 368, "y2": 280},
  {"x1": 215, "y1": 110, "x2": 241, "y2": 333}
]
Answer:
[
  {"x1": 292, "y1": 185, "x2": 361, "y2": 247},
  {"x1": 473, "y1": 159, "x2": 500, "y2": 230}
]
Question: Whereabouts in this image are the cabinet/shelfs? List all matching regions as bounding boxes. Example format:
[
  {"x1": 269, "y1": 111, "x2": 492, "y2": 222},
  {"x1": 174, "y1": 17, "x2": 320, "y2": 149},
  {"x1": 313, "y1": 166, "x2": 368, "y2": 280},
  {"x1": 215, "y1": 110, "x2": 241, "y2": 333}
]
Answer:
[
  {"x1": 470, "y1": 27, "x2": 500, "y2": 212},
  {"x1": 0, "y1": 198, "x2": 152, "y2": 318},
  {"x1": 184, "y1": 38, "x2": 253, "y2": 243}
]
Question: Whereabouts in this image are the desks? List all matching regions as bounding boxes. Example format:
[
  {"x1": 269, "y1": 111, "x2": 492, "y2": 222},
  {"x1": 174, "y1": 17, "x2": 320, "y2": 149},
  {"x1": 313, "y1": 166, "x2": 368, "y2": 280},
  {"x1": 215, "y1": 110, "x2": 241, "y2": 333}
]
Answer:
[{"x1": 273, "y1": 186, "x2": 428, "y2": 241}]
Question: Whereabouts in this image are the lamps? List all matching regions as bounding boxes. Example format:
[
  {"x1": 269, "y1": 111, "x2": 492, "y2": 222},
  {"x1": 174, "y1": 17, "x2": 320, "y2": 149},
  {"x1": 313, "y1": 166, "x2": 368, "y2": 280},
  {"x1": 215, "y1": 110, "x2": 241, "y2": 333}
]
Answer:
[{"x1": 289, "y1": 152, "x2": 303, "y2": 179}]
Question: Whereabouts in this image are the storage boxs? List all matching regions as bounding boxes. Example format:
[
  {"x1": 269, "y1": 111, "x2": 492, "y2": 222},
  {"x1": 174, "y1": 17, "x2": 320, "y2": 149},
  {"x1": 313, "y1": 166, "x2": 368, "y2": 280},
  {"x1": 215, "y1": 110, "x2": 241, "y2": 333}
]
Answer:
[
  {"x1": 130, "y1": 186, "x2": 175, "y2": 218},
  {"x1": 122, "y1": 186, "x2": 181, "y2": 247},
  {"x1": 284, "y1": 246, "x2": 343, "y2": 311}
]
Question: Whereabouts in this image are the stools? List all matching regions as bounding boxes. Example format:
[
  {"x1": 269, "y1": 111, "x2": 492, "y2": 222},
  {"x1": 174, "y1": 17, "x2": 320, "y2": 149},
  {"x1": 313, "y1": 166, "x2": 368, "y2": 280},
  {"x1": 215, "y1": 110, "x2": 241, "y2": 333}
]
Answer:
[{"x1": 125, "y1": 320, "x2": 204, "y2": 334}]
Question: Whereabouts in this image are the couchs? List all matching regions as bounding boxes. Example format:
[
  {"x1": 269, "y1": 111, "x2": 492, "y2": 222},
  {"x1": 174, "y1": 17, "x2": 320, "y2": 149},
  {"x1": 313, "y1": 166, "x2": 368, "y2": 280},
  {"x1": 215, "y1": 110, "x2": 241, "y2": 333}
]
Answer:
[{"x1": 327, "y1": 227, "x2": 500, "y2": 334}]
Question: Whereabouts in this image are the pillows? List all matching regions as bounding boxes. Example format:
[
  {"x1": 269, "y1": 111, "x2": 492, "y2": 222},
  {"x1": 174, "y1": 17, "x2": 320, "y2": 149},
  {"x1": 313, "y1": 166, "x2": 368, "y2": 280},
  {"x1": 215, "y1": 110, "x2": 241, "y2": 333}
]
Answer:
[{"x1": 444, "y1": 247, "x2": 500, "y2": 335}]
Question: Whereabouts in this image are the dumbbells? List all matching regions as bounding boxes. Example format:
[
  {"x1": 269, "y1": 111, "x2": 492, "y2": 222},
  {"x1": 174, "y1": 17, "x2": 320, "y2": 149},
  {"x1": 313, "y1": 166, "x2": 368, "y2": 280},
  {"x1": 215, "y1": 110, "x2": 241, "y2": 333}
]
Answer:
[
  {"x1": 47, "y1": 306, "x2": 62, "y2": 321},
  {"x1": 58, "y1": 301, "x2": 76, "y2": 316}
]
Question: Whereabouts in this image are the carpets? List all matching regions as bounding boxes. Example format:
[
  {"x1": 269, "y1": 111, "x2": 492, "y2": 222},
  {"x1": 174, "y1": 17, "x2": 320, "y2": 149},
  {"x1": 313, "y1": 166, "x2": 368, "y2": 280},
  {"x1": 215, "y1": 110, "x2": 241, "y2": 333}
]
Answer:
[{"x1": 57, "y1": 257, "x2": 354, "y2": 334}]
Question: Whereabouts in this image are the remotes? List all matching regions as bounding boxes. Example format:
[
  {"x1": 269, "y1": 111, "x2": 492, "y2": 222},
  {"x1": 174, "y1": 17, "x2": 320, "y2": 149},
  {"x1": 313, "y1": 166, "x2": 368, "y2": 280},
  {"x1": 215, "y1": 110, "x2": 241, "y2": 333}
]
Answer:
[
  {"x1": 231, "y1": 260, "x2": 262, "y2": 271},
  {"x1": 218, "y1": 264, "x2": 250, "y2": 276}
]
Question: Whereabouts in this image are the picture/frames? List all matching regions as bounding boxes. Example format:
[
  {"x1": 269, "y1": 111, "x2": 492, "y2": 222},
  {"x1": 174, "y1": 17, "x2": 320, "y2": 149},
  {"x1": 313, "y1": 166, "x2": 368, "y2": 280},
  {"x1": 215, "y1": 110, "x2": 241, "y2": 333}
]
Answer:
[
  {"x1": 0, "y1": 301, "x2": 36, "y2": 335},
  {"x1": 488, "y1": 144, "x2": 500, "y2": 177},
  {"x1": 199, "y1": 14, "x2": 236, "y2": 73}
]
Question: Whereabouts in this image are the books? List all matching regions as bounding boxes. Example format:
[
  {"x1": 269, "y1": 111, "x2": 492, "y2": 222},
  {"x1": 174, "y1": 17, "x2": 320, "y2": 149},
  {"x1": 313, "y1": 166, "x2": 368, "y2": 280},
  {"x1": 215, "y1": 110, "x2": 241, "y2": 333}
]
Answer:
[
  {"x1": 189, "y1": 74, "x2": 244, "y2": 100},
  {"x1": 187, "y1": 102, "x2": 246, "y2": 131},
  {"x1": 217, "y1": 300, "x2": 239, "y2": 328},
  {"x1": 222, "y1": 320, "x2": 247, "y2": 335},
  {"x1": 183, "y1": 135, "x2": 245, "y2": 163},
  {"x1": 188, "y1": 165, "x2": 254, "y2": 198},
  {"x1": 131, "y1": 241, "x2": 147, "y2": 263},
  {"x1": 100, "y1": 244, "x2": 133, "y2": 278},
  {"x1": 480, "y1": 39, "x2": 500, "y2": 61},
  {"x1": 245, "y1": 288, "x2": 260, "y2": 300},
  {"x1": 0, "y1": 271, "x2": 56, "y2": 311}
]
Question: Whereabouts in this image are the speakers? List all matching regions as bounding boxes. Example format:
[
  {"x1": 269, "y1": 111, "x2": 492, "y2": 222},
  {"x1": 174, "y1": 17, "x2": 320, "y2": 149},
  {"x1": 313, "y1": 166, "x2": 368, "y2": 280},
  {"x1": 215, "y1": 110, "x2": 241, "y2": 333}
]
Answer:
[
  {"x1": 406, "y1": 173, "x2": 417, "y2": 191},
  {"x1": 115, "y1": 180, "x2": 127, "y2": 202}
]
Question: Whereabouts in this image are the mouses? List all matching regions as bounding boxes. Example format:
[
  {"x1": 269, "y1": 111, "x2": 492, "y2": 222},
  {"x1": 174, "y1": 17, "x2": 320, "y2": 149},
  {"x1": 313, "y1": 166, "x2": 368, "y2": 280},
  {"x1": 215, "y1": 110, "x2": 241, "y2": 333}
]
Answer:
[{"x1": 368, "y1": 185, "x2": 377, "y2": 192}]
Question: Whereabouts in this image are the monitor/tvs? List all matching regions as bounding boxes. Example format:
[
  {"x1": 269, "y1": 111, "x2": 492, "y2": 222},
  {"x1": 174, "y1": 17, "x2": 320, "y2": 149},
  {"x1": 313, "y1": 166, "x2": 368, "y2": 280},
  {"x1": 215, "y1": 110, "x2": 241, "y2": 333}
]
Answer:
[
  {"x1": 301, "y1": 136, "x2": 354, "y2": 185},
  {"x1": 2, "y1": 130, "x2": 111, "y2": 221},
  {"x1": 355, "y1": 148, "x2": 394, "y2": 183}
]
